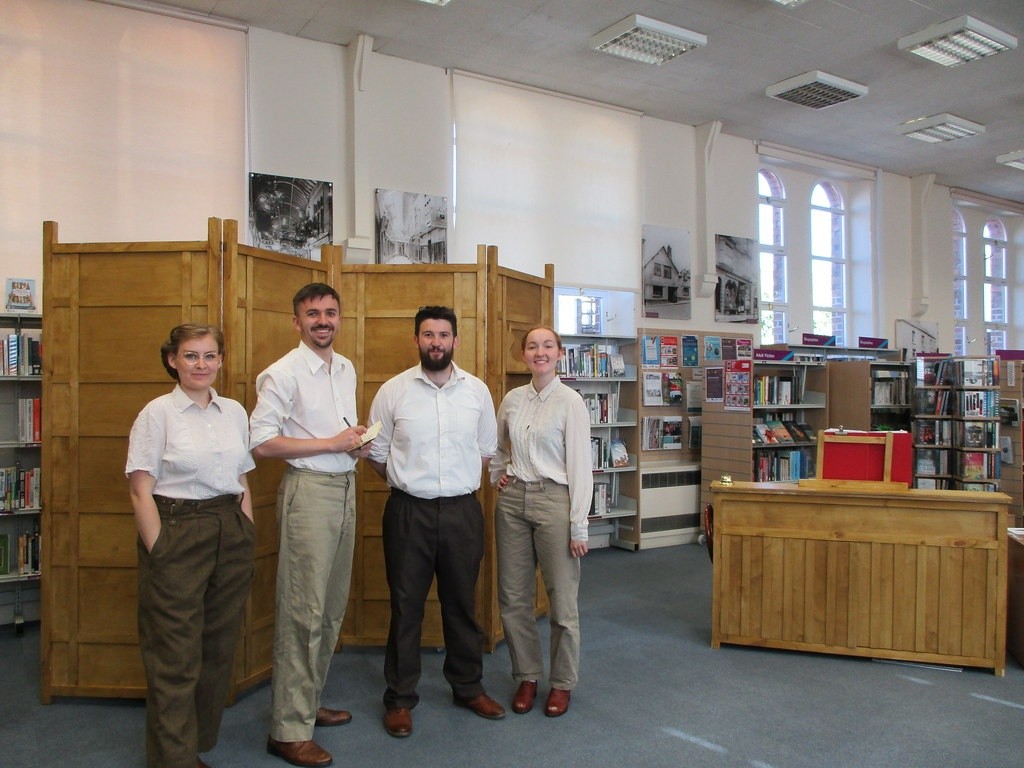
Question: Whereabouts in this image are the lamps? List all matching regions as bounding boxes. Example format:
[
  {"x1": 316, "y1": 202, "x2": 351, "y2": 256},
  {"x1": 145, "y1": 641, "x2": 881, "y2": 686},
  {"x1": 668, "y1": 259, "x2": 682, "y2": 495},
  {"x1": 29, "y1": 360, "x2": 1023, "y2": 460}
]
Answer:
[
  {"x1": 896, "y1": 15, "x2": 1018, "y2": 69},
  {"x1": 995, "y1": 150, "x2": 1024, "y2": 171},
  {"x1": 766, "y1": 71, "x2": 868, "y2": 111},
  {"x1": 897, "y1": 113, "x2": 986, "y2": 144},
  {"x1": 589, "y1": 14, "x2": 706, "y2": 65}
]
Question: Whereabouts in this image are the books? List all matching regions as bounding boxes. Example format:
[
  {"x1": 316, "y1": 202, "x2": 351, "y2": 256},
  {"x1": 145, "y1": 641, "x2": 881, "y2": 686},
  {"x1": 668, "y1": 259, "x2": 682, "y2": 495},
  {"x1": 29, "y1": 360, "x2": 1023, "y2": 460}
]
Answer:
[
  {"x1": 0, "y1": 334, "x2": 42, "y2": 577},
  {"x1": 556, "y1": 341, "x2": 1024, "y2": 516},
  {"x1": 347, "y1": 420, "x2": 382, "y2": 452}
]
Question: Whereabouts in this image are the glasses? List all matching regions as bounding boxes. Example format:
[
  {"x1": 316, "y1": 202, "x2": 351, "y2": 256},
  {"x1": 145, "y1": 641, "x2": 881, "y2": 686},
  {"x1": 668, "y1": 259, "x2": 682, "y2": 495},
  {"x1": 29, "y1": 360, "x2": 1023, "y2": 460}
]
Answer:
[{"x1": 178, "y1": 351, "x2": 221, "y2": 366}]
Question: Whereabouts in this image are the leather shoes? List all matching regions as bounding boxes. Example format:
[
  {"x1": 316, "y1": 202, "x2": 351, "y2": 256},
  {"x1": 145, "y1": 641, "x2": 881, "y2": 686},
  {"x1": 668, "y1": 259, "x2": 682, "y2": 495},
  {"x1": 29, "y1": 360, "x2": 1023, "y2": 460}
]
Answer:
[
  {"x1": 512, "y1": 679, "x2": 538, "y2": 713},
  {"x1": 454, "y1": 691, "x2": 505, "y2": 719},
  {"x1": 545, "y1": 688, "x2": 570, "y2": 717},
  {"x1": 266, "y1": 734, "x2": 332, "y2": 768},
  {"x1": 314, "y1": 706, "x2": 353, "y2": 727},
  {"x1": 384, "y1": 706, "x2": 412, "y2": 737}
]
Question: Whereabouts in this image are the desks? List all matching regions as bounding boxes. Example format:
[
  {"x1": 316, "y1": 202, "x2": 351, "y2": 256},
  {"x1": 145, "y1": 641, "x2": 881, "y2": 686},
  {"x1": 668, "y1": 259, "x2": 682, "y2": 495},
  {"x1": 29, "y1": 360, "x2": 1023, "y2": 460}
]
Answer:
[{"x1": 708, "y1": 480, "x2": 1013, "y2": 678}]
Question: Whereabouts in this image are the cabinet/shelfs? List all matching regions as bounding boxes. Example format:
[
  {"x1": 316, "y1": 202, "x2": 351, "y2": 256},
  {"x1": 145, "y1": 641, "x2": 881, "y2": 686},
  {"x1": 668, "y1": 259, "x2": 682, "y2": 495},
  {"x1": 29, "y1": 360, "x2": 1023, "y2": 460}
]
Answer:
[
  {"x1": 0, "y1": 311, "x2": 42, "y2": 635},
  {"x1": 556, "y1": 328, "x2": 1024, "y2": 551}
]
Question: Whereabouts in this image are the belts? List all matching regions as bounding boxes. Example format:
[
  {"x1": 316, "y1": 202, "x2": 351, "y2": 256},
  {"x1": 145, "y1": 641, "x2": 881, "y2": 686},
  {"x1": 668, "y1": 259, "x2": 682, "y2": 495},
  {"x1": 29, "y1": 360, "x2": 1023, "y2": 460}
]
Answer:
[{"x1": 157, "y1": 493, "x2": 237, "y2": 513}]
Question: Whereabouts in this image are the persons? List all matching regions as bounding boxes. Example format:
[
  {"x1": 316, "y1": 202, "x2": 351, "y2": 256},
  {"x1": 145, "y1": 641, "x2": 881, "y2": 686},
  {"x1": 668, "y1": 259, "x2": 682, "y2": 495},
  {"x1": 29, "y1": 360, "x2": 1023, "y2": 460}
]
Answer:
[
  {"x1": 490, "y1": 326, "x2": 594, "y2": 716},
  {"x1": 125, "y1": 323, "x2": 256, "y2": 768},
  {"x1": 249, "y1": 284, "x2": 373, "y2": 768},
  {"x1": 369, "y1": 304, "x2": 505, "y2": 740}
]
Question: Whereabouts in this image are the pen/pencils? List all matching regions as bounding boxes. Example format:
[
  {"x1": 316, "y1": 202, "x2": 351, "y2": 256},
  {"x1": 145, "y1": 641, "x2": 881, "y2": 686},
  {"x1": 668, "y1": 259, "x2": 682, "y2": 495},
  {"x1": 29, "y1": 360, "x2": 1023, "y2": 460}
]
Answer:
[{"x1": 343, "y1": 416, "x2": 351, "y2": 428}]
